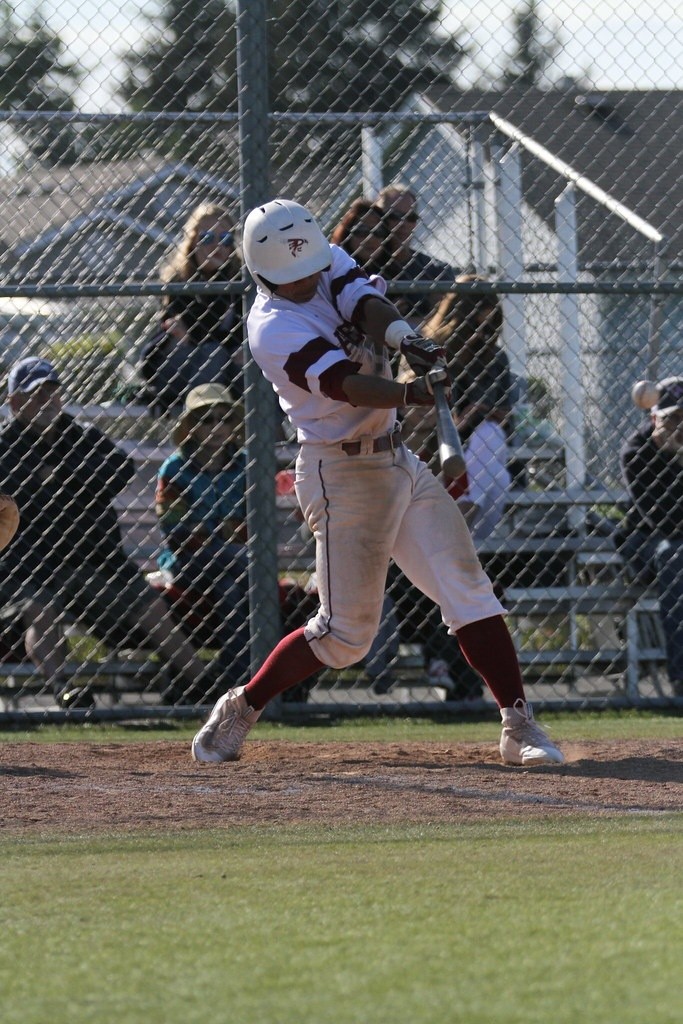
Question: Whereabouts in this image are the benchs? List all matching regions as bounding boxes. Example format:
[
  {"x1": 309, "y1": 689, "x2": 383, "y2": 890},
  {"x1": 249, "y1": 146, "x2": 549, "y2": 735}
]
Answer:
[{"x1": 0, "y1": 404, "x2": 673, "y2": 718}]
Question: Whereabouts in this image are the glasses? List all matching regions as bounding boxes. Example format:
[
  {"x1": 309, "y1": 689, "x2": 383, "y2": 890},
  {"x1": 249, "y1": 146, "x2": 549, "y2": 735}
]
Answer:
[
  {"x1": 346, "y1": 224, "x2": 390, "y2": 241},
  {"x1": 386, "y1": 210, "x2": 420, "y2": 224},
  {"x1": 193, "y1": 230, "x2": 233, "y2": 247},
  {"x1": 193, "y1": 413, "x2": 234, "y2": 425}
]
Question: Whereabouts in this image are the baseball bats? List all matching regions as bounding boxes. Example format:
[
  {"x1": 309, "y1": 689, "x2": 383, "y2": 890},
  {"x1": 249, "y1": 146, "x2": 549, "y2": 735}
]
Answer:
[{"x1": 430, "y1": 366, "x2": 467, "y2": 481}]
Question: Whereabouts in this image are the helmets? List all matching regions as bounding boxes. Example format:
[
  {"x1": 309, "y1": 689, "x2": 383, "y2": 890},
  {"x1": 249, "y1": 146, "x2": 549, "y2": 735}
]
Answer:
[{"x1": 243, "y1": 199, "x2": 334, "y2": 295}]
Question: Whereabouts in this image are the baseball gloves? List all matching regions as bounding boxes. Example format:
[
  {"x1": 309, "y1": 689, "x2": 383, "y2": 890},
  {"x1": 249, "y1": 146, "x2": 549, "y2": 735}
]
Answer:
[{"x1": 0, "y1": 491, "x2": 20, "y2": 553}]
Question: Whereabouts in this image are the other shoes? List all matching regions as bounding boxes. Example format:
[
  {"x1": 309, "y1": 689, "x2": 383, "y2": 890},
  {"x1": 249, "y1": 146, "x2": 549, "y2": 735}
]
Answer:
[
  {"x1": 187, "y1": 672, "x2": 229, "y2": 702},
  {"x1": 50, "y1": 675, "x2": 94, "y2": 712}
]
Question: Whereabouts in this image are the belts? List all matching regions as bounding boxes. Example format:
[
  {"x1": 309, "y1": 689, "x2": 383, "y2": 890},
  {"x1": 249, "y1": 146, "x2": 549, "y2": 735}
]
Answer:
[{"x1": 341, "y1": 424, "x2": 402, "y2": 456}]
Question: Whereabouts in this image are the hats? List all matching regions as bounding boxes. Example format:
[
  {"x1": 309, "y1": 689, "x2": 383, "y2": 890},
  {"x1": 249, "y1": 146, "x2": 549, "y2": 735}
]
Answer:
[
  {"x1": 650, "y1": 375, "x2": 683, "y2": 417},
  {"x1": 6, "y1": 356, "x2": 62, "y2": 401},
  {"x1": 173, "y1": 383, "x2": 244, "y2": 451}
]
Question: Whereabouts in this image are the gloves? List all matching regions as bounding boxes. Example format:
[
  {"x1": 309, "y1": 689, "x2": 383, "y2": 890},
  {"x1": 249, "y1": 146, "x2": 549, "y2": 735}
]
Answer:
[
  {"x1": 401, "y1": 367, "x2": 452, "y2": 407},
  {"x1": 385, "y1": 321, "x2": 452, "y2": 378}
]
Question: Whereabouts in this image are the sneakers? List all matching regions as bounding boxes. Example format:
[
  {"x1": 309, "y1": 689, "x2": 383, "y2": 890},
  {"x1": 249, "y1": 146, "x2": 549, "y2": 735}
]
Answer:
[
  {"x1": 191, "y1": 685, "x2": 266, "y2": 763},
  {"x1": 498, "y1": 699, "x2": 563, "y2": 768}
]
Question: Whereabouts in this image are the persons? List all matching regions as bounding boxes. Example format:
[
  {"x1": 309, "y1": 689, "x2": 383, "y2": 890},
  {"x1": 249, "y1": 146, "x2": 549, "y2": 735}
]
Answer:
[
  {"x1": 329, "y1": 187, "x2": 455, "y2": 332},
  {"x1": 140, "y1": 205, "x2": 245, "y2": 404},
  {"x1": 614, "y1": 375, "x2": 683, "y2": 699},
  {"x1": 365, "y1": 276, "x2": 514, "y2": 702},
  {"x1": 0, "y1": 357, "x2": 220, "y2": 708},
  {"x1": 190, "y1": 200, "x2": 571, "y2": 765},
  {"x1": 154, "y1": 383, "x2": 321, "y2": 705}
]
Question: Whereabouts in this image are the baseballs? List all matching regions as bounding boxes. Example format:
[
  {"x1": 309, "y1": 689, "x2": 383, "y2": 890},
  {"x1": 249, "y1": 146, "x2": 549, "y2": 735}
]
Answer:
[{"x1": 631, "y1": 381, "x2": 660, "y2": 408}]
